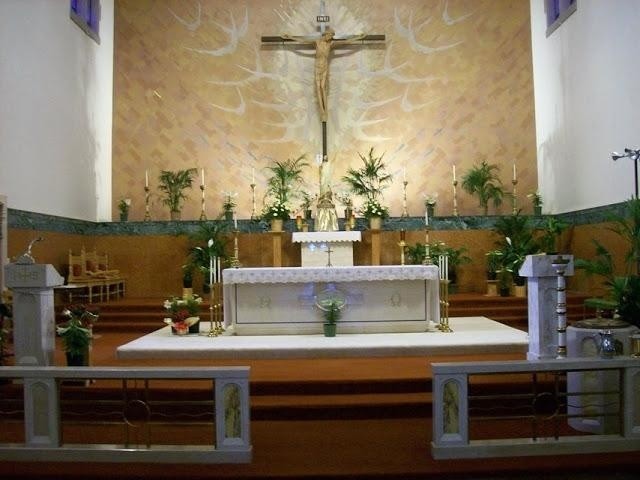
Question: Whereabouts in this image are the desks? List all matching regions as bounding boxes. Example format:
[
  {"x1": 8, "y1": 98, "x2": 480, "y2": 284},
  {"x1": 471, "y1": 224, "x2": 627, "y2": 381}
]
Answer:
[{"x1": 221, "y1": 264, "x2": 434, "y2": 335}]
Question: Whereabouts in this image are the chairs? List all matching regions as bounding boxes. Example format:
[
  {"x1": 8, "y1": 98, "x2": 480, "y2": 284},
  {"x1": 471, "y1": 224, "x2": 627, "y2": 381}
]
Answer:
[
  {"x1": 66, "y1": 247, "x2": 128, "y2": 302},
  {"x1": 582, "y1": 276, "x2": 630, "y2": 318}
]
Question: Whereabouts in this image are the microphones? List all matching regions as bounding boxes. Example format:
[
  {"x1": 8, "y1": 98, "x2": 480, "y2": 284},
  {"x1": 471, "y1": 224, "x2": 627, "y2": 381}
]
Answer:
[{"x1": 26, "y1": 236, "x2": 45, "y2": 255}]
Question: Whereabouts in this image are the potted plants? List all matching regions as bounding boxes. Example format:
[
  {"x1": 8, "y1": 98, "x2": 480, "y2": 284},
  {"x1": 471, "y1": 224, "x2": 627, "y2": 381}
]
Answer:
[
  {"x1": 160, "y1": 165, "x2": 236, "y2": 334},
  {"x1": 118, "y1": 198, "x2": 131, "y2": 222},
  {"x1": 56, "y1": 309, "x2": 94, "y2": 366},
  {"x1": 340, "y1": 149, "x2": 393, "y2": 231},
  {"x1": 410, "y1": 162, "x2": 560, "y2": 287},
  {"x1": 314, "y1": 298, "x2": 345, "y2": 337},
  {"x1": 261, "y1": 153, "x2": 309, "y2": 231}
]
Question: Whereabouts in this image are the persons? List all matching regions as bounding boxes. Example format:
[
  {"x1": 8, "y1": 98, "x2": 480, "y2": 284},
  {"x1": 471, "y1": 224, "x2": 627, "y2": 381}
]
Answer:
[
  {"x1": 280, "y1": 28, "x2": 368, "y2": 121},
  {"x1": 319, "y1": 152, "x2": 337, "y2": 198}
]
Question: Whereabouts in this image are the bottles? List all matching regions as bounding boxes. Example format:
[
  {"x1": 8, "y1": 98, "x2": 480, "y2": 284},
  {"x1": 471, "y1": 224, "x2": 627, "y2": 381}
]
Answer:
[{"x1": 597, "y1": 332, "x2": 615, "y2": 358}]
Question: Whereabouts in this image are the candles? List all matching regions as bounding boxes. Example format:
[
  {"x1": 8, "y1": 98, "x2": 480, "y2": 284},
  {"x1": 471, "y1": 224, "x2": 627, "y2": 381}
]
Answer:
[
  {"x1": 145, "y1": 169, "x2": 149, "y2": 187},
  {"x1": 252, "y1": 167, "x2": 255, "y2": 185}
]
